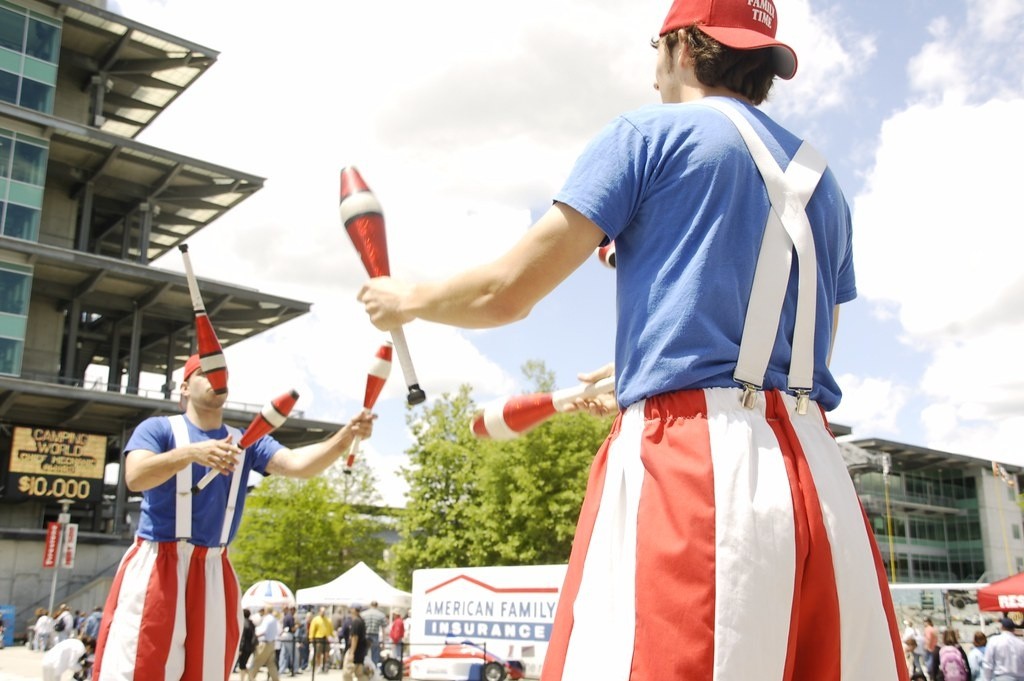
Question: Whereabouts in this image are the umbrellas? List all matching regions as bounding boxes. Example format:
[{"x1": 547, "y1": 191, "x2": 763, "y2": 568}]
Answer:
[{"x1": 241, "y1": 580, "x2": 295, "y2": 608}]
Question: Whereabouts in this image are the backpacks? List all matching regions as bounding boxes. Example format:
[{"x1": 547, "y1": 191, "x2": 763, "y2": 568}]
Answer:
[{"x1": 938, "y1": 646, "x2": 968, "y2": 681}]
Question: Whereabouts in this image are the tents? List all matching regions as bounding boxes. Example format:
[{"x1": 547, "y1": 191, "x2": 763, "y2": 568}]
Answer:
[
  {"x1": 295, "y1": 561, "x2": 412, "y2": 614},
  {"x1": 976, "y1": 571, "x2": 1024, "y2": 632}
]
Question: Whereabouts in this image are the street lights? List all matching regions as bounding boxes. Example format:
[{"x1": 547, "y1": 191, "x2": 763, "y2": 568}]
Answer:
[{"x1": 48, "y1": 498, "x2": 76, "y2": 615}]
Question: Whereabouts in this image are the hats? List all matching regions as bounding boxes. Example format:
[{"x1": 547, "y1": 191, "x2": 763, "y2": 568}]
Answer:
[
  {"x1": 185, "y1": 354, "x2": 204, "y2": 381},
  {"x1": 659, "y1": 0, "x2": 798, "y2": 80},
  {"x1": 391, "y1": 608, "x2": 400, "y2": 615},
  {"x1": 998, "y1": 618, "x2": 1014, "y2": 630}
]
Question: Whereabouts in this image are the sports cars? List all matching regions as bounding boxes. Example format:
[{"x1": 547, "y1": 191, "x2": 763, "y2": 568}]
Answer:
[{"x1": 379, "y1": 638, "x2": 524, "y2": 681}]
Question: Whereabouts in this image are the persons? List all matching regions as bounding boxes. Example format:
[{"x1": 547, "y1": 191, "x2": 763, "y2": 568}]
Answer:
[
  {"x1": 356, "y1": 0, "x2": 908, "y2": 681},
  {"x1": 27, "y1": 604, "x2": 104, "y2": 681},
  {"x1": 94, "y1": 354, "x2": 378, "y2": 681},
  {"x1": 233, "y1": 601, "x2": 411, "y2": 681},
  {"x1": 901, "y1": 616, "x2": 1024, "y2": 681}
]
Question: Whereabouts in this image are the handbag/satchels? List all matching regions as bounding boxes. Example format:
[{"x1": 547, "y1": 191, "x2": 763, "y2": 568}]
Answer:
[{"x1": 53, "y1": 618, "x2": 65, "y2": 631}]
